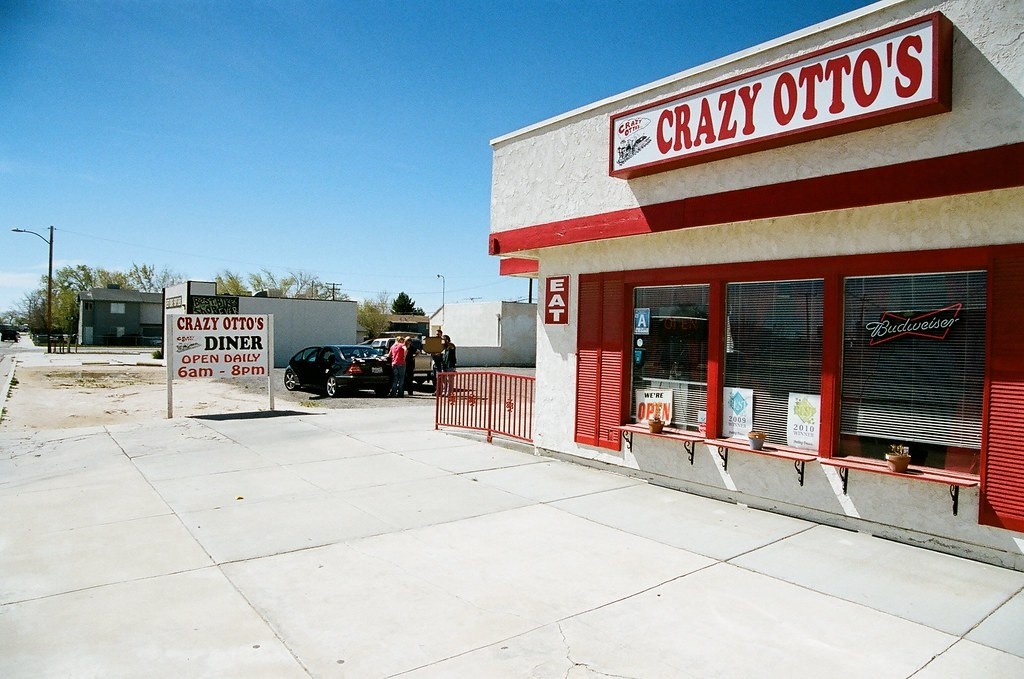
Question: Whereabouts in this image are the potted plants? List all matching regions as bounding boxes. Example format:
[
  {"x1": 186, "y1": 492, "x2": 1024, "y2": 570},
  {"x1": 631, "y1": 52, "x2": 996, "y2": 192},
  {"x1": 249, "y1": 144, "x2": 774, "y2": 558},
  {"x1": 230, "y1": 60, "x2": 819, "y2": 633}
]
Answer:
[
  {"x1": 885, "y1": 444, "x2": 911, "y2": 473},
  {"x1": 648, "y1": 400, "x2": 665, "y2": 433}
]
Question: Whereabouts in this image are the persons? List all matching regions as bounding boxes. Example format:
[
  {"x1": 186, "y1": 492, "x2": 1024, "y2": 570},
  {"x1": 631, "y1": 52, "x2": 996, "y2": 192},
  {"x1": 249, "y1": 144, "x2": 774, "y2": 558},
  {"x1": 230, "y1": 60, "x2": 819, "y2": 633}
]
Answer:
[
  {"x1": 383, "y1": 336, "x2": 409, "y2": 398},
  {"x1": 441, "y1": 335, "x2": 456, "y2": 398},
  {"x1": 430, "y1": 329, "x2": 445, "y2": 396},
  {"x1": 403, "y1": 336, "x2": 421, "y2": 396}
]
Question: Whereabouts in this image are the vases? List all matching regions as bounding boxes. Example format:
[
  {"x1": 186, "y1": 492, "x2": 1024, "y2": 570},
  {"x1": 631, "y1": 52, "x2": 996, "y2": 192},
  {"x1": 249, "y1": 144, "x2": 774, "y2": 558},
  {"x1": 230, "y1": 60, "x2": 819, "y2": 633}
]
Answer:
[{"x1": 747, "y1": 431, "x2": 766, "y2": 450}]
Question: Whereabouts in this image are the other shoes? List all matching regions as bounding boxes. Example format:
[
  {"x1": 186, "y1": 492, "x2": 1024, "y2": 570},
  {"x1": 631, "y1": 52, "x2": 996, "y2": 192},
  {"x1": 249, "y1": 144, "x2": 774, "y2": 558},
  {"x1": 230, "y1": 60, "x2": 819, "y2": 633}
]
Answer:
[
  {"x1": 386, "y1": 393, "x2": 396, "y2": 398},
  {"x1": 396, "y1": 393, "x2": 405, "y2": 398},
  {"x1": 432, "y1": 391, "x2": 437, "y2": 396}
]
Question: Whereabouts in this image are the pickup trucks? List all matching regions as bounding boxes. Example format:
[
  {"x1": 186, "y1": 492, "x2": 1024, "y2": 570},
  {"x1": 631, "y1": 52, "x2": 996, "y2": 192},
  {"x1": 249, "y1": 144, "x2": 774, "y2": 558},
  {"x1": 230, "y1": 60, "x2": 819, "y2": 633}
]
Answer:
[{"x1": 359, "y1": 338, "x2": 436, "y2": 384}]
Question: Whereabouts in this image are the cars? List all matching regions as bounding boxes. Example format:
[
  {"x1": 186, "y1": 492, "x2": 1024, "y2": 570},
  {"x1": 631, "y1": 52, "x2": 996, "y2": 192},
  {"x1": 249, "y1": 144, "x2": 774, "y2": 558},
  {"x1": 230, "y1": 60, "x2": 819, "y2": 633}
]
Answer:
[
  {"x1": 144, "y1": 337, "x2": 162, "y2": 346},
  {"x1": 1, "y1": 329, "x2": 18, "y2": 343},
  {"x1": 284, "y1": 342, "x2": 396, "y2": 397}
]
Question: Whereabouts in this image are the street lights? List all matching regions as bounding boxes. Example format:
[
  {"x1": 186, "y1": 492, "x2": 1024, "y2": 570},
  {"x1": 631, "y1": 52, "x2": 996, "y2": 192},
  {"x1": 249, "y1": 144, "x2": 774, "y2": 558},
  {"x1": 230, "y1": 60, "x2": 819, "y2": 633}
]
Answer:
[
  {"x1": 11, "y1": 225, "x2": 54, "y2": 352},
  {"x1": 436, "y1": 274, "x2": 445, "y2": 325}
]
